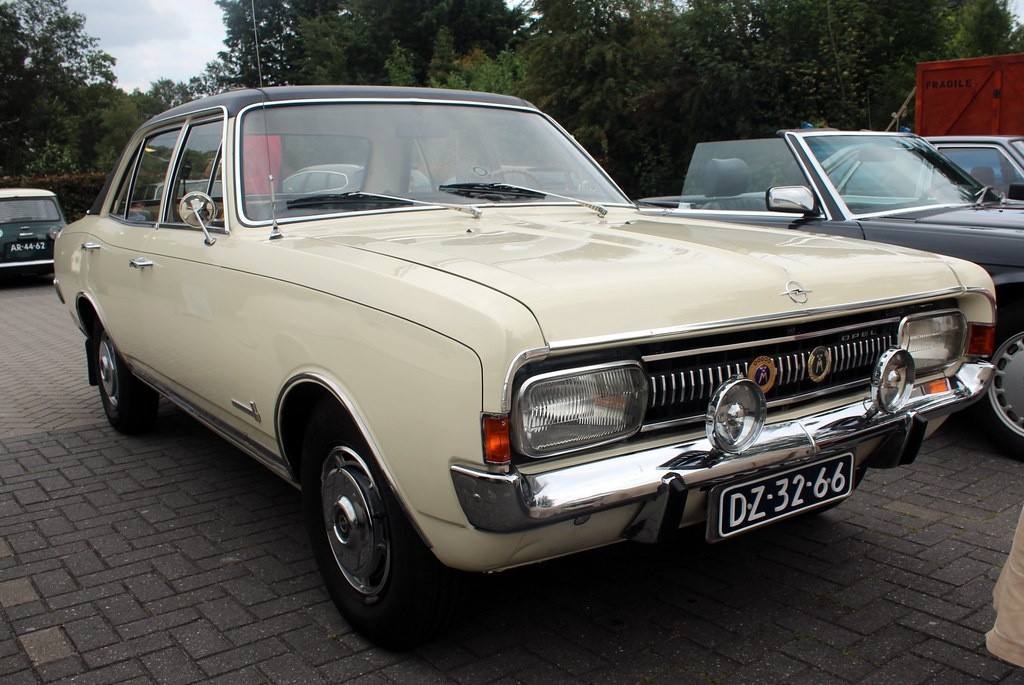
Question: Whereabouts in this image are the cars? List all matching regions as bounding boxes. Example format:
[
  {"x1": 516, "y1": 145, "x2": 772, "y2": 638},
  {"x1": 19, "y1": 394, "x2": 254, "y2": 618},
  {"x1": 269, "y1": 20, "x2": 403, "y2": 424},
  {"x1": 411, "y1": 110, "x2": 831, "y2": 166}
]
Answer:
[
  {"x1": 820, "y1": 136, "x2": 1024, "y2": 207},
  {"x1": 442, "y1": 165, "x2": 581, "y2": 197},
  {"x1": 136, "y1": 179, "x2": 225, "y2": 202},
  {"x1": 52, "y1": 86, "x2": 1000, "y2": 651},
  {"x1": 280, "y1": 163, "x2": 437, "y2": 199},
  {"x1": 637, "y1": 125, "x2": 1024, "y2": 463},
  {"x1": 0, "y1": 188, "x2": 65, "y2": 286}
]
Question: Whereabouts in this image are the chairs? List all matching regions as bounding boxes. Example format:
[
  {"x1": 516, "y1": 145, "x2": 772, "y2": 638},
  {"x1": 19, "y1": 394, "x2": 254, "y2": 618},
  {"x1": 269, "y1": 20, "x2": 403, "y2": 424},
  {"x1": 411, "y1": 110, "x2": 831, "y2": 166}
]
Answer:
[
  {"x1": 970, "y1": 165, "x2": 996, "y2": 192},
  {"x1": 697, "y1": 157, "x2": 770, "y2": 214}
]
